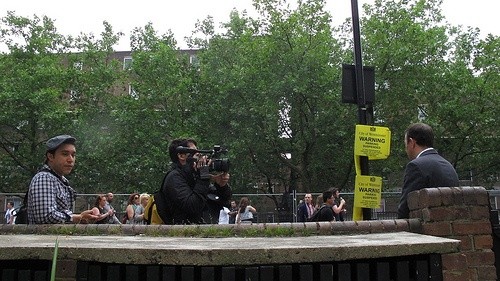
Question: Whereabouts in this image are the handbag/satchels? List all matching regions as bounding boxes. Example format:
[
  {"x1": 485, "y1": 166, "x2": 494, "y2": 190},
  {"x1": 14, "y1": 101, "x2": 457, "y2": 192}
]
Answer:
[
  {"x1": 11, "y1": 202, "x2": 29, "y2": 224},
  {"x1": 122, "y1": 215, "x2": 129, "y2": 224}
]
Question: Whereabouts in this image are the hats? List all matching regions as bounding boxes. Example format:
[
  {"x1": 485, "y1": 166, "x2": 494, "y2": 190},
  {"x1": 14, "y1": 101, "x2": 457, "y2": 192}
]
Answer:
[{"x1": 46, "y1": 135, "x2": 77, "y2": 153}]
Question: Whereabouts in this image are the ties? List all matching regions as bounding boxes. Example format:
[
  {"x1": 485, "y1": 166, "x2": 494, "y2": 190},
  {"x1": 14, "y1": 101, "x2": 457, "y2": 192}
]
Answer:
[{"x1": 307, "y1": 204, "x2": 311, "y2": 218}]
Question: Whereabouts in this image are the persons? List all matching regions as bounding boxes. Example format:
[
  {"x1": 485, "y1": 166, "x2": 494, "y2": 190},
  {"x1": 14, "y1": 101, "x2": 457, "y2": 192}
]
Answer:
[
  {"x1": 134, "y1": 193, "x2": 150, "y2": 225},
  {"x1": 296, "y1": 189, "x2": 347, "y2": 222},
  {"x1": 143, "y1": 194, "x2": 163, "y2": 226},
  {"x1": 125, "y1": 193, "x2": 140, "y2": 225},
  {"x1": 218, "y1": 201, "x2": 236, "y2": 224},
  {"x1": 236, "y1": 197, "x2": 257, "y2": 225},
  {"x1": 26, "y1": 135, "x2": 103, "y2": 224},
  {"x1": 4, "y1": 202, "x2": 17, "y2": 225},
  {"x1": 90, "y1": 196, "x2": 114, "y2": 225},
  {"x1": 228, "y1": 202, "x2": 238, "y2": 223},
  {"x1": 162, "y1": 138, "x2": 233, "y2": 225},
  {"x1": 102, "y1": 192, "x2": 122, "y2": 224},
  {"x1": 396, "y1": 122, "x2": 460, "y2": 219}
]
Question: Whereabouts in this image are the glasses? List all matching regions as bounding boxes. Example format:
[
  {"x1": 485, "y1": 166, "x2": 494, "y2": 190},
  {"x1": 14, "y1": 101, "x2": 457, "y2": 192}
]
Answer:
[{"x1": 134, "y1": 197, "x2": 139, "y2": 199}]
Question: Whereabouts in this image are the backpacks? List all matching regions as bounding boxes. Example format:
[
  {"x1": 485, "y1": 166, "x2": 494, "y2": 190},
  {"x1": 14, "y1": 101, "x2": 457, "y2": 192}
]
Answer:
[{"x1": 310, "y1": 205, "x2": 325, "y2": 222}]
